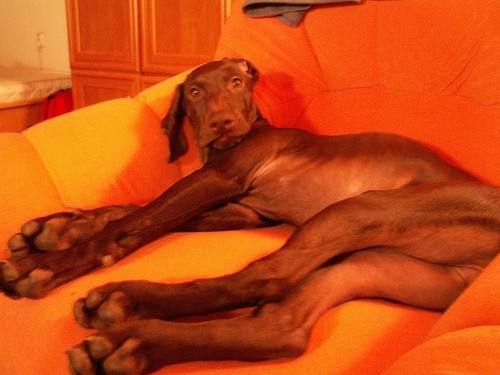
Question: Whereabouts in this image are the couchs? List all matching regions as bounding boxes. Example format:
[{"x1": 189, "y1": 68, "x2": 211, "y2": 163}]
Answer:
[{"x1": 1, "y1": 0, "x2": 500, "y2": 375}]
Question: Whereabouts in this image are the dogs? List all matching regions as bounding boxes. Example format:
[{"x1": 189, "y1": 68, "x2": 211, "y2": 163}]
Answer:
[{"x1": 1, "y1": 57, "x2": 495, "y2": 374}]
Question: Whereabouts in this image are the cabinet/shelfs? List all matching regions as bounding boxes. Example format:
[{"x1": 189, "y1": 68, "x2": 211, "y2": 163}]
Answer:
[{"x1": 66, "y1": 0, "x2": 230, "y2": 109}]
